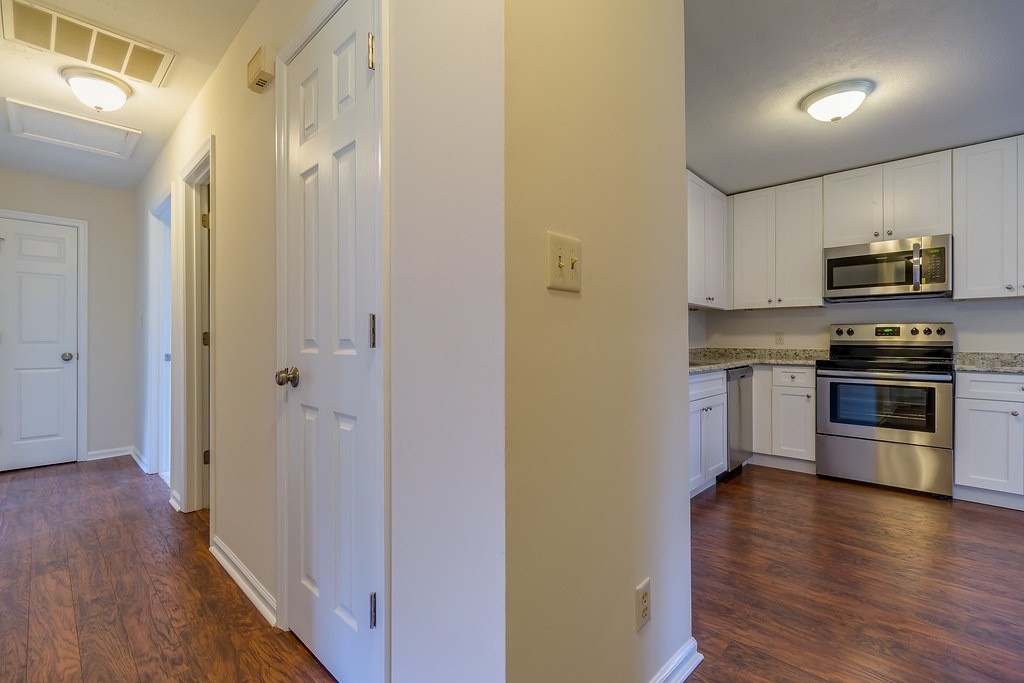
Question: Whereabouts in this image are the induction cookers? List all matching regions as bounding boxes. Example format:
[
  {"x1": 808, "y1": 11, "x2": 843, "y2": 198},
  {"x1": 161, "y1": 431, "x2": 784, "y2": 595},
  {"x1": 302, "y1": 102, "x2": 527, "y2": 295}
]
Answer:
[{"x1": 817, "y1": 322, "x2": 954, "y2": 381}]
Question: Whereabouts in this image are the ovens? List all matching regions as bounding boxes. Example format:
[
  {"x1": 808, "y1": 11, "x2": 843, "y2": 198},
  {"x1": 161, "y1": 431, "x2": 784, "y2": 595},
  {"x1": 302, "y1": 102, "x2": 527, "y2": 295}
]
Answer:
[{"x1": 816, "y1": 376, "x2": 954, "y2": 449}]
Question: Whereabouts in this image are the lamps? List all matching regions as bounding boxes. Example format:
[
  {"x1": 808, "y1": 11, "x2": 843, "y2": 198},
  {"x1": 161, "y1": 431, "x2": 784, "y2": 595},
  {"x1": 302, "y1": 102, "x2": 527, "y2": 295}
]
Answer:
[
  {"x1": 61, "y1": 66, "x2": 133, "y2": 113},
  {"x1": 799, "y1": 78, "x2": 875, "y2": 124}
]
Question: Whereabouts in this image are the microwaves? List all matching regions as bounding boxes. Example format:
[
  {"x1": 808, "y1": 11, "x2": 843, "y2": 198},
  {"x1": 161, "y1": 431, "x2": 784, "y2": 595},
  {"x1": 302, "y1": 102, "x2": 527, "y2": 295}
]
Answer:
[{"x1": 822, "y1": 233, "x2": 953, "y2": 305}]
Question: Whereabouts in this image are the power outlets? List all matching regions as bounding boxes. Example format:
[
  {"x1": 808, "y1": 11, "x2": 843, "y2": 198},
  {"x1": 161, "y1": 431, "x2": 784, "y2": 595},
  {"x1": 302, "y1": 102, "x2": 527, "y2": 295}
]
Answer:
[
  {"x1": 776, "y1": 331, "x2": 784, "y2": 345},
  {"x1": 635, "y1": 577, "x2": 652, "y2": 632}
]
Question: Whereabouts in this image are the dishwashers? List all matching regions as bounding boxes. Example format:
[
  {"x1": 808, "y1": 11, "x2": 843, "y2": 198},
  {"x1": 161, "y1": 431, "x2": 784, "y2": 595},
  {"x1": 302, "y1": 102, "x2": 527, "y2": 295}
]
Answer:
[{"x1": 726, "y1": 366, "x2": 754, "y2": 472}]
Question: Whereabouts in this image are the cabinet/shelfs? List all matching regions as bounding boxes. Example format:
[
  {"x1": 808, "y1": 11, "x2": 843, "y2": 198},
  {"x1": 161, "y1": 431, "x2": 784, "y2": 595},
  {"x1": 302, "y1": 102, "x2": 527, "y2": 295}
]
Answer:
[
  {"x1": 686, "y1": 370, "x2": 729, "y2": 499},
  {"x1": 726, "y1": 366, "x2": 753, "y2": 472},
  {"x1": 741, "y1": 363, "x2": 816, "y2": 476},
  {"x1": 686, "y1": 133, "x2": 1024, "y2": 310},
  {"x1": 953, "y1": 370, "x2": 1024, "y2": 512}
]
